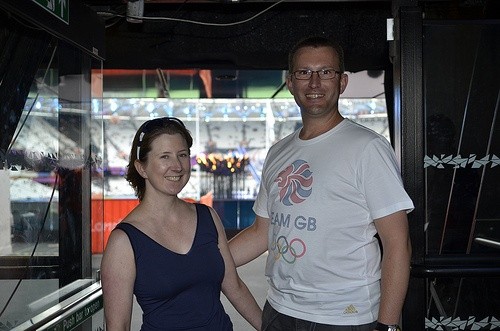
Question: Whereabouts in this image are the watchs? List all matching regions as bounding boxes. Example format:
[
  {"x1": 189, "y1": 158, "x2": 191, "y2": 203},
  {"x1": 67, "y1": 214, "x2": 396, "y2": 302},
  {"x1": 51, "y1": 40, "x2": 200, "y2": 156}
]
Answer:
[{"x1": 374, "y1": 321, "x2": 402, "y2": 331}]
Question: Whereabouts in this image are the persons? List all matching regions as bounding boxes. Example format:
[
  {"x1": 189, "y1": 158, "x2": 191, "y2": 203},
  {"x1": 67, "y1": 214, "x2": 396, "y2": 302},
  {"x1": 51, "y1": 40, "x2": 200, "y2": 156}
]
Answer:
[
  {"x1": 98, "y1": 116, "x2": 263, "y2": 331},
  {"x1": 227, "y1": 33, "x2": 416, "y2": 331}
]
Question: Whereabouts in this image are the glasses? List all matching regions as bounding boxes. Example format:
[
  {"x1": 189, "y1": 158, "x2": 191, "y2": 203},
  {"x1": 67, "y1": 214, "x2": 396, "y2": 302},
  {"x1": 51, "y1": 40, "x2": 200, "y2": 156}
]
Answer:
[{"x1": 291, "y1": 69, "x2": 341, "y2": 79}]
